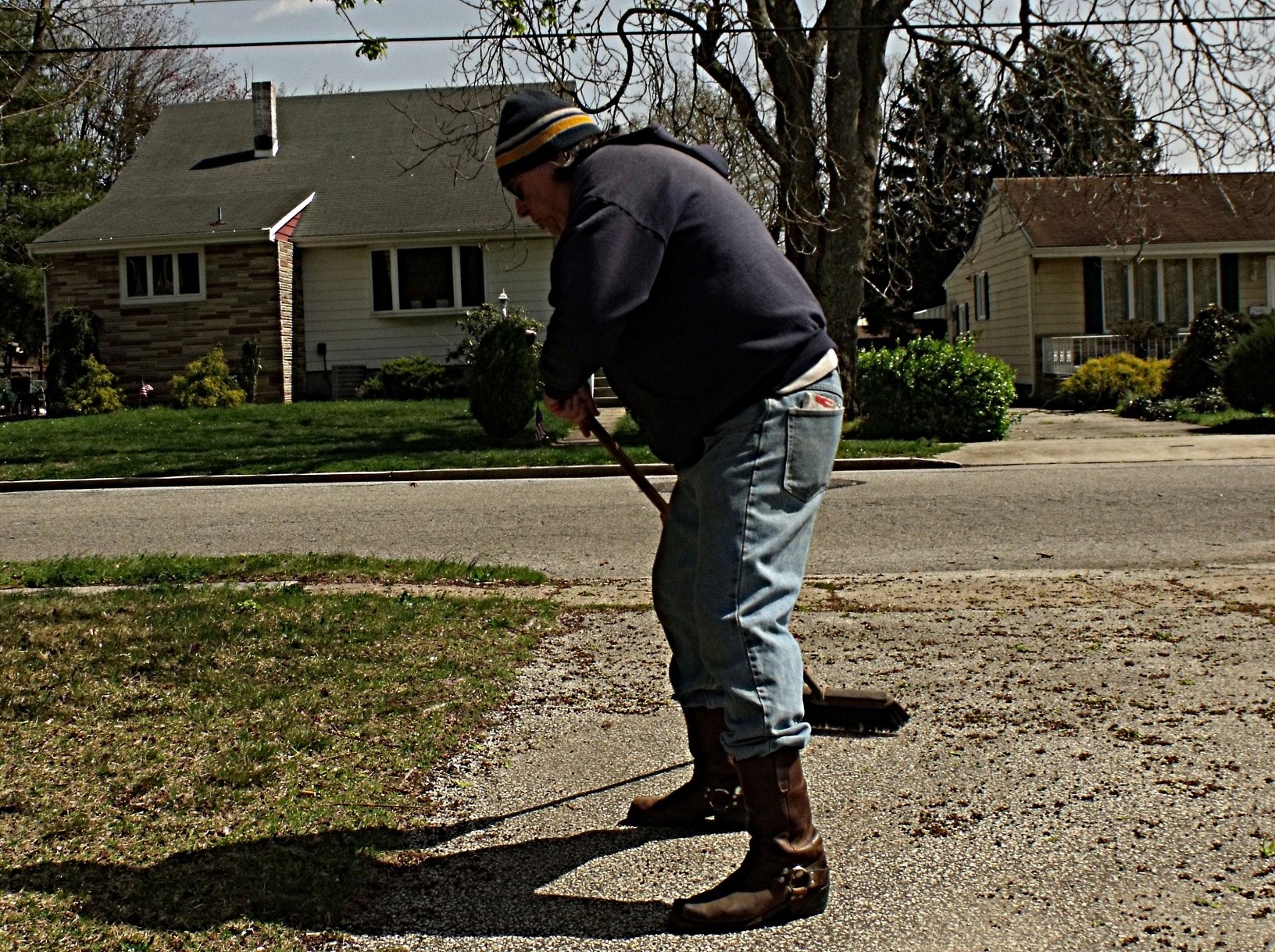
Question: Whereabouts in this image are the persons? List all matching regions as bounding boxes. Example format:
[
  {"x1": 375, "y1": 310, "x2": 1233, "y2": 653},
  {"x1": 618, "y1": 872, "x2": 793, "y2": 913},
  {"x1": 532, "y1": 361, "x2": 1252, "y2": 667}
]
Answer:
[{"x1": 495, "y1": 89, "x2": 846, "y2": 932}]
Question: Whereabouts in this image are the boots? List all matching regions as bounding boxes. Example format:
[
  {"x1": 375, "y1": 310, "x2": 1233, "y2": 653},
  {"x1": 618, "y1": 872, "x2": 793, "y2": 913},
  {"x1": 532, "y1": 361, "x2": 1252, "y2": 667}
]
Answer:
[
  {"x1": 629, "y1": 704, "x2": 748, "y2": 824},
  {"x1": 671, "y1": 747, "x2": 831, "y2": 932}
]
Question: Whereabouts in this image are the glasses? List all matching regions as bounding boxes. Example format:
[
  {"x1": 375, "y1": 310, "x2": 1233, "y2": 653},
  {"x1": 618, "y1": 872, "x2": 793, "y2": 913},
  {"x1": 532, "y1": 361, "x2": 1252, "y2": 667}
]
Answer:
[{"x1": 502, "y1": 175, "x2": 525, "y2": 203}]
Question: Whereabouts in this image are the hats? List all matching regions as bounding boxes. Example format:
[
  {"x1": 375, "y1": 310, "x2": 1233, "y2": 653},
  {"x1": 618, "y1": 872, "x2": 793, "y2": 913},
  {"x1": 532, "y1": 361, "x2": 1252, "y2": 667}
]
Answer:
[{"x1": 493, "y1": 89, "x2": 602, "y2": 184}]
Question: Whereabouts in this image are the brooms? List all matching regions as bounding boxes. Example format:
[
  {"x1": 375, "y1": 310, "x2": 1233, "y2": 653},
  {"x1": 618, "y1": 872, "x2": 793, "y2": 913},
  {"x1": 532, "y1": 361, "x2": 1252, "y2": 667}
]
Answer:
[{"x1": 579, "y1": 409, "x2": 909, "y2": 735}]
